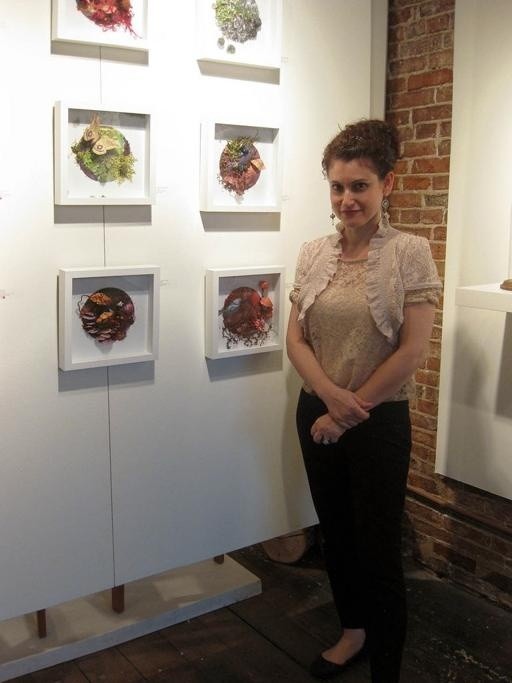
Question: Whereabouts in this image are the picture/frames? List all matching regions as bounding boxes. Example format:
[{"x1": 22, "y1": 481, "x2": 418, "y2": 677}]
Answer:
[
  {"x1": 199, "y1": 123, "x2": 284, "y2": 213},
  {"x1": 197, "y1": 1, "x2": 281, "y2": 69},
  {"x1": 54, "y1": 100, "x2": 156, "y2": 206},
  {"x1": 59, "y1": 266, "x2": 159, "y2": 371},
  {"x1": 50, "y1": 0, "x2": 149, "y2": 53},
  {"x1": 206, "y1": 266, "x2": 285, "y2": 360}
]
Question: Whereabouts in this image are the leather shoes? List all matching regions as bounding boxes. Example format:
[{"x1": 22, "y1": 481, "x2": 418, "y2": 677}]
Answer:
[{"x1": 309, "y1": 649, "x2": 349, "y2": 678}]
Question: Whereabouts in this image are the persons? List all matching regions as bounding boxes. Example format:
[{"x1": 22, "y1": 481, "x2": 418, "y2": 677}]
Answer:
[{"x1": 284, "y1": 117, "x2": 441, "y2": 681}]
